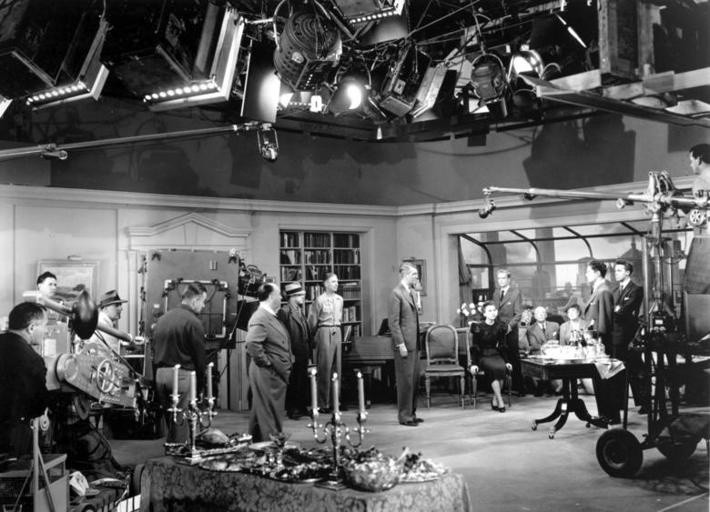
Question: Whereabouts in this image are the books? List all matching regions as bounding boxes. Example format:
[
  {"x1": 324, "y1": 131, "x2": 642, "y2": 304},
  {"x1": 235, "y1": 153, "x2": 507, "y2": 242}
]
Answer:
[{"x1": 280, "y1": 229, "x2": 361, "y2": 342}]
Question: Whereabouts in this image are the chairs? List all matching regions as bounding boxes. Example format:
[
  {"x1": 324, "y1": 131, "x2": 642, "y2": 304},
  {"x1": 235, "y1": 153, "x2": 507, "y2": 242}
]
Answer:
[{"x1": 422, "y1": 322, "x2": 513, "y2": 408}]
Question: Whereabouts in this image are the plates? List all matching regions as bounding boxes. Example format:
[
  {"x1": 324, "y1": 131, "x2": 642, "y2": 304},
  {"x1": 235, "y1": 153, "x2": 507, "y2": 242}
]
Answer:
[{"x1": 199, "y1": 429, "x2": 453, "y2": 495}]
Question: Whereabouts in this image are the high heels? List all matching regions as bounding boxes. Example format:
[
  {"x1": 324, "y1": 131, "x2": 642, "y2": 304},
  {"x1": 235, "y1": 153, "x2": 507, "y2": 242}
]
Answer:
[
  {"x1": 491, "y1": 399, "x2": 498, "y2": 410},
  {"x1": 498, "y1": 406, "x2": 505, "y2": 412}
]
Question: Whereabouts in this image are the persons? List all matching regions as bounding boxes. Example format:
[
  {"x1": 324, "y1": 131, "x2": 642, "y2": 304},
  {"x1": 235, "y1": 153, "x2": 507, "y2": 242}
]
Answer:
[
  {"x1": 527, "y1": 304, "x2": 559, "y2": 397},
  {"x1": 306, "y1": 270, "x2": 345, "y2": 413},
  {"x1": 386, "y1": 259, "x2": 427, "y2": 430},
  {"x1": 469, "y1": 299, "x2": 510, "y2": 411},
  {"x1": 492, "y1": 266, "x2": 525, "y2": 394},
  {"x1": 607, "y1": 257, "x2": 647, "y2": 416},
  {"x1": 556, "y1": 304, "x2": 588, "y2": 396},
  {"x1": 0, "y1": 302, "x2": 96, "y2": 483},
  {"x1": 276, "y1": 280, "x2": 313, "y2": 422},
  {"x1": 150, "y1": 281, "x2": 209, "y2": 452},
  {"x1": 585, "y1": 259, "x2": 623, "y2": 427},
  {"x1": 242, "y1": 282, "x2": 297, "y2": 454},
  {"x1": 85, "y1": 288, "x2": 124, "y2": 354},
  {"x1": 674, "y1": 144, "x2": 710, "y2": 298},
  {"x1": 26, "y1": 271, "x2": 66, "y2": 325}
]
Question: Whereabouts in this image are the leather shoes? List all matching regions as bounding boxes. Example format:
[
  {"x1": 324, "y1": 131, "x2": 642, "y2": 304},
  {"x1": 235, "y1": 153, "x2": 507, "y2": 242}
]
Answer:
[{"x1": 400, "y1": 418, "x2": 424, "y2": 426}]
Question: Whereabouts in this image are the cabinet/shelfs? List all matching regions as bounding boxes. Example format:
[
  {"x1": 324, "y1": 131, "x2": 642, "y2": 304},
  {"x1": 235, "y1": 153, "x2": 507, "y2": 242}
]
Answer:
[{"x1": 277, "y1": 228, "x2": 362, "y2": 355}]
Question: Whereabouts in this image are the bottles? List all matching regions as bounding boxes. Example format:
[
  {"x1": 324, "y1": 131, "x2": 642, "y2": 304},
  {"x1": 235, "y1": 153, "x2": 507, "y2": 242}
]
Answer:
[{"x1": 540, "y1": 327, "x2": 609, "y2": 364}]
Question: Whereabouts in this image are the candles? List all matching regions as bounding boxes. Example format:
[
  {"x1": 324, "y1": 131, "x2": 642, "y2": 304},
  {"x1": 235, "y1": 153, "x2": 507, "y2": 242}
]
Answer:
[
  {"x1": 306, "y1": 376, "x2": 364, "y2": 414},
  {"x1": 172, "y1": 364, "x2": 215, "y2": 401}
]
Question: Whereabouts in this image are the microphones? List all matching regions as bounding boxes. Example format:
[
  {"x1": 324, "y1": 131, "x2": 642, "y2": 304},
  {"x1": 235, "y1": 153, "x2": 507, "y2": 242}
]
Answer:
[{"x1": 480, "y1": 203, "x2": 497, "y2": 219}]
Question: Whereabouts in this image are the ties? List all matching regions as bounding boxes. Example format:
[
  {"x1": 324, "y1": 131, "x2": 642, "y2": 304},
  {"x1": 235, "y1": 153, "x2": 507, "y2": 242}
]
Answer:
[
  {"x1": 500, "y1": 290, "x2": 504, "y2": 301},
  {"x1": 620, "y1": 285, "x2": 623, "y2": 296},
  {"x1": 542, "y1": 324, "x2": 545, "y2": 335}
]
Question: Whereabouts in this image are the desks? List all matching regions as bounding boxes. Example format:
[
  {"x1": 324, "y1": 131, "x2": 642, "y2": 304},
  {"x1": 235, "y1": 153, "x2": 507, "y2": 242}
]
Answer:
[{"x1": 521, "y1": 356, "x2": 609, "y2": 438}]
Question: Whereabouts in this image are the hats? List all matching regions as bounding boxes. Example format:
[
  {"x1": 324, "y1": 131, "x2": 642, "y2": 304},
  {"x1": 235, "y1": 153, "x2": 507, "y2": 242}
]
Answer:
[
  {"x1": 285, "y1": 284, "x2": 306, "y2": 297},
  {"x1": 100, "y1": 290, "x2": 128, "y2": 309}
]
